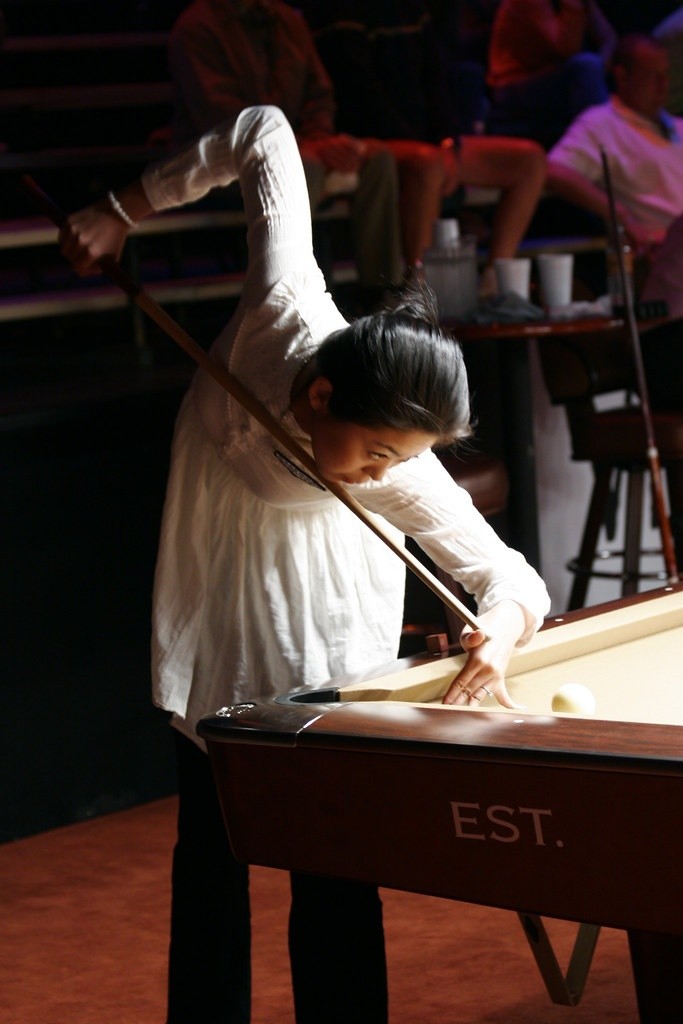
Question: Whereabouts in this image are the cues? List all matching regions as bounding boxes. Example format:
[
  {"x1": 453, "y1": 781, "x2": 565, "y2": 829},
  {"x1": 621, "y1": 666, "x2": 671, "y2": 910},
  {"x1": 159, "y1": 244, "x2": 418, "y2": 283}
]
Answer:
[
  {"x1": 600, "y1": 150, "x2": 678, "y2": 583},
  {"x1": 15, "y1": 172, "x2": 480, "y2": 632}
]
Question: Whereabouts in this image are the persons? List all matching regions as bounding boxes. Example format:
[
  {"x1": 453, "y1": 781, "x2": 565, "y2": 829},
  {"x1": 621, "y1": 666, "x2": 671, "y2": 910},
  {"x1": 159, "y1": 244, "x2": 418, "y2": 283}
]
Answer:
[
  {"x1": 57, "y1": 104, "x2": 551, "y2": 1024},
  {"x1": 169, "y1": 0, "x2": 682, "y2": 327},
  {"x1": 626, "y1": 215, "x2": 683, "y2": 586}
]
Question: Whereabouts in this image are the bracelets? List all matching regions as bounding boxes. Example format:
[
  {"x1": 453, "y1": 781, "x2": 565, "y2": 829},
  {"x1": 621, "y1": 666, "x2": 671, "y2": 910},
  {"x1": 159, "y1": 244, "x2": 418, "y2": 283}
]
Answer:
[{"x1": 108, "y1": 191, "x2": 139, "y2": 228}]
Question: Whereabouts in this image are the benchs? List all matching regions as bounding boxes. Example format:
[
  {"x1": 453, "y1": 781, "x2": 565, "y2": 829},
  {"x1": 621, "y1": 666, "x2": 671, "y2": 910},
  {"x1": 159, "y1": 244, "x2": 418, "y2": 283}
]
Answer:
[{"x1": 2, "y1": 1, "x2": 665, "y2": 374}]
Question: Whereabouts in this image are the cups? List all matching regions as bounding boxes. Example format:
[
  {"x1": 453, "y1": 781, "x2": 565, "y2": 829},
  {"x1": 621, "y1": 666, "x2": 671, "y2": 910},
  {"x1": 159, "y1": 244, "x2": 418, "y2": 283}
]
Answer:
[
  {"x1": 497, "y1": 257, "x2": 530, "y2": 299},
  {"x1": 540, "y1": 253, "x2": 574, "y2": 307}
]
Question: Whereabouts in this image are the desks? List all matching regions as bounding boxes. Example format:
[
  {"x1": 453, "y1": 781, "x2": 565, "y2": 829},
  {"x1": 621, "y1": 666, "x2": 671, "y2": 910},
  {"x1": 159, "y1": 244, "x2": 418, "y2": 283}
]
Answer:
[
  {"x1": 402, "y1": 307, "x2": 660, "y2": 617},
  {"x1": 196, "y1": 578, "x2": 683, "y2": 1024}
]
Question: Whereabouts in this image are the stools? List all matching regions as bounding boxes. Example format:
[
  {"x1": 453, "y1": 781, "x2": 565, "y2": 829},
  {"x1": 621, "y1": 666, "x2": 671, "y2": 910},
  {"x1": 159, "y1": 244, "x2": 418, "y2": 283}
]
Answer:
[
  {"x1": 567, "y1": 405, "x2": 683, "y2": 610},
  {"x1": 404, "y1": 453, "x2": 514, "y2": 651}
]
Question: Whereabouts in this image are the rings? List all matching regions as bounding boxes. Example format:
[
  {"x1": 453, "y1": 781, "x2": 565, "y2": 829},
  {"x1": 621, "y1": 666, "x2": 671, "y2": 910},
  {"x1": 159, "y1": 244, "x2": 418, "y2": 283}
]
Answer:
[{"x1": 481, "y1": 686, "x2": 494, "y2": 697}]
